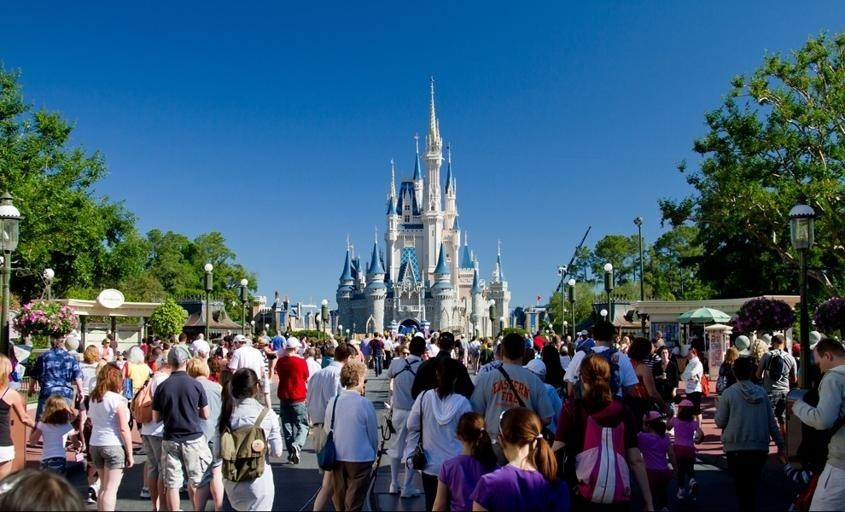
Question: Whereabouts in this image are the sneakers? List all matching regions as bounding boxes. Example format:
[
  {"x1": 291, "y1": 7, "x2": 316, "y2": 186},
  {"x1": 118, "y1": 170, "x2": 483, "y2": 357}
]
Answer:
[
  {"x1": 140, "y1": 488, "x2": 152, "y2": 498},
  {"x1": 289, "y1": 443, "x2": 302, "y2": 465},
  {"x1": 676, "y1": 479, "x2": 698, "y2": 500},
  {"x1": 389, "y1": 481, "x2": 421, "y2": 498},
  {"x1": 88, "y1": 487, "x2": 99, "y2": 504}
]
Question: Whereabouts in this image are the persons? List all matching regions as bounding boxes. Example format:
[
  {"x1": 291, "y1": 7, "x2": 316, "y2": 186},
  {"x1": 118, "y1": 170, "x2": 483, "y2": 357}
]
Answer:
[
  {"x1": 0, "y1": 467, "x2": 88, "y2": 511},
  {"x1": 0, "y1": 353, "x2": 29, "y2": 487}
]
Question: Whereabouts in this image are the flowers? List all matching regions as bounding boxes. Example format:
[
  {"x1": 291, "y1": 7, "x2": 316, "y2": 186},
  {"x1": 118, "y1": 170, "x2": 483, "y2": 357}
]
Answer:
[
  {"x1": 736, "y1": 297, "x2": 795, "y2": 333},
  {"x1": 12, "y1": 301, "x2": 83, "y2": 338},
  {"x1": 813, "y1": 295, "x2": 845, "y2": 334}
]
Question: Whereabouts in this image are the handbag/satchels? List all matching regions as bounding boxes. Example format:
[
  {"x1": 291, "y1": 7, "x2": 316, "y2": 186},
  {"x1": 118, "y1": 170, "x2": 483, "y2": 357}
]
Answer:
[
  {"x1": 317, "y1": 431, "x2": 337, "y2": 470},
  {"x1": 404, "y1": 442, "x2": 428, "y2": 470},
  {"x1": 122, "y1": 362, "x2": 133, "y2": 400},
  {"x1": 131, "y1": 372, "x2": 154, "y2": 424}
]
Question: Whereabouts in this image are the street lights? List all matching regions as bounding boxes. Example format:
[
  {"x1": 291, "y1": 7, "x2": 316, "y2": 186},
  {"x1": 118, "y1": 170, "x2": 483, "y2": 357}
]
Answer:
[
  {"x1": 338, "y1": 324, "x2": 342, "y2": 338},
  {"x1": 321, "y1": 298, "x2": 329, "y2": 333},
  {"x1": 346, "y1": 328, "x2": 350, "y2": 342},
  {"x1": 569, "y1": 278, "x2": 577, "y2": 340},
  {"x1": 40, "y1": 266, "x2": 56, "y2": 350},
  {"x1": 200, "y1": 262, "x2": 214, "y2": 341},
  {"x1": 238, "y1": 277, "x2": 248, "y2": 336},
  {"x1": 603, "y1": 262, "x2": 615, "y2": 323},
  {"x1": 786, "y1": 203, "x2": 819, "y2": 481},
  {"x1": 633, "y1": 214, "x2": 648, "y2": 303},
  {"x1": 488, "y1": 297, "x2": 497, "y2": 341},
  {"x1": 0, "y1": 187, "x2": 25, "y2": 359}
]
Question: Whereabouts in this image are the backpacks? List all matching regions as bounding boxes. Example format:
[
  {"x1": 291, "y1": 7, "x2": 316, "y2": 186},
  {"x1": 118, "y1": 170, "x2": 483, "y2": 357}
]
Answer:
[
  {"x1": 766, "y1": 354, "x2": 790, "y2": 382},
  {"x1": 220, "y1": 406, "x2": 271, "y2": 481},
  {"x1": 701, "y1": 375, "x2": 709, "y2": 397},
  {"x1": 582, "y1": 348, "x2": 620, "y2": 395},
  {"x1": 575, "y1": 401, "x2": 631, "y2": 504}
]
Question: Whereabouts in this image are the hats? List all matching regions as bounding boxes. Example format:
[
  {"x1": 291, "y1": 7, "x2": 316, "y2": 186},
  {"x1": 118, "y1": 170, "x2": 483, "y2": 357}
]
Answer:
[
  {"x1": 167, "y1": 347, "x2": 188, "y2": 364},
  {"x1": 674, "y1": 399, "x2": 694, "y2": 407},
  {"x1": 286, "y1": 337, "x2": 302, "y2": 347},
  {"x1": 534, "y1": 335, "x2": 545, "y2": 350},
  {"x1": 642, "y1": 411, "x2": 661, "y2": 421},
  {"x1": 582, "y1": 330, "x2": 589, "y2": 335},
  {"x1": 233, "y1": 335, "x2": 247, "y2": 342}
]
